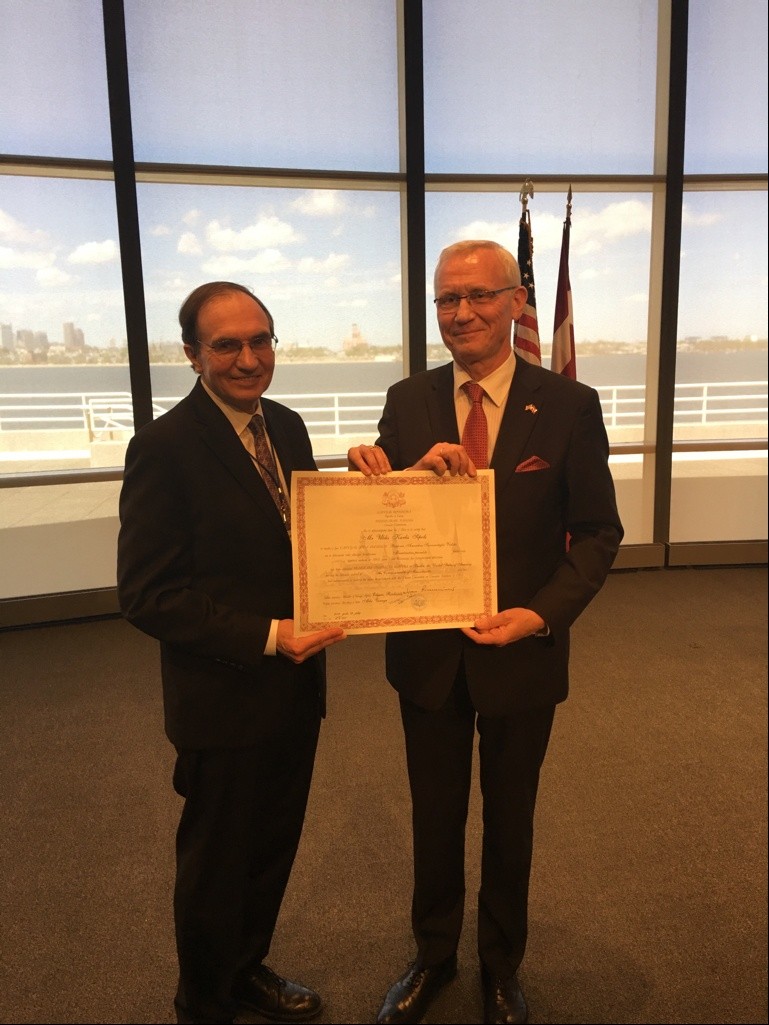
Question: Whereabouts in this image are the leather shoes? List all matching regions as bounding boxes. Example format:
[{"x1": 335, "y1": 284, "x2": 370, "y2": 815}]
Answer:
[
  {"x1": 479, "y1": 962, "x2": 529, "y2": 1025},
  {"x1": 376, "y1": 955, "x2": 458, "y2": 1025},
  {"x1": 240, "y1": 963, "x2": 324, "y2": 1021}
]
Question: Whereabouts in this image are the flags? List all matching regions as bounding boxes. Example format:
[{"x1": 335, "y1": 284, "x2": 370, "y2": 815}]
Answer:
[
  {"x1": 551, "y1": 217, "x2": 576, "y2": 379},
  {"x1": 513, "y1": 211, "x2": 542, "y2": 367}
]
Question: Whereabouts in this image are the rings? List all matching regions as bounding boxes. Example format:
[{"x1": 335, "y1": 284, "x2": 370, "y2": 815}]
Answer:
[
  {"x1": 371, "y1": 446, "x2": 376, "y2": 450},
  {"x1": 440, "y1": 446, "x2": 444, "y2": 456}
]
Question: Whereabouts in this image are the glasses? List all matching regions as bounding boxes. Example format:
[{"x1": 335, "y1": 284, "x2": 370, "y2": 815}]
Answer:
[
  {"x1": 197, "y1": 334, "x2": 279, "y2": 358},
  {"x1": 434, "y1": 285, "x2": 518, "y2": 311}
]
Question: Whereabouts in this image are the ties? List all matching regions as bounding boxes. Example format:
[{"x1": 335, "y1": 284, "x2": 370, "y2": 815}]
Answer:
[
  {"x1": 248, "y1": 414, "x2": 291, "y2": 545},
  {"x1": 461, "y1": 381, "x2": 489, "y2": 470}
]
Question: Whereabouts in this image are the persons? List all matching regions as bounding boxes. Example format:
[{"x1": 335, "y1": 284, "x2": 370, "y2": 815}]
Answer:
[
  {"x1": 374, "y1": 239, "x2": 624, "y2": 1025},
  {"x1": 116, "y1": 281, "x2": 391, "y2": 1025}
]
